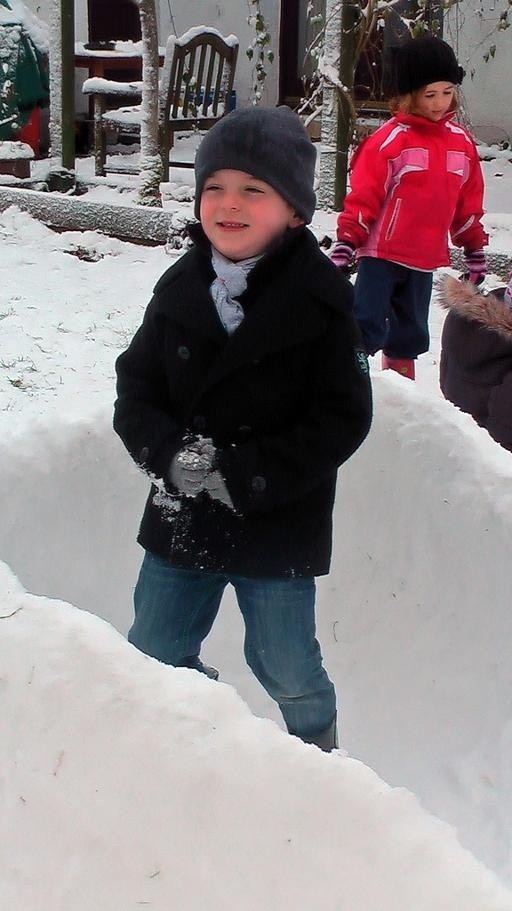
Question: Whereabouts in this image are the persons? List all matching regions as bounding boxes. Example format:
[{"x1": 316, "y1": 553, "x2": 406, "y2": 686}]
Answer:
[
  {"x1": 334, "y1": 32, "x2": 489, "y2": 381},
  {"x1": 108, "y1": 106, "x2": 373, "y2": 759}
]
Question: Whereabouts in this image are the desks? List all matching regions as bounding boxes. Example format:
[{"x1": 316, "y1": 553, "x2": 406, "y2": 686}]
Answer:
[{"x1": 74, "y1": 56, "x2": 167, "y2": 157}]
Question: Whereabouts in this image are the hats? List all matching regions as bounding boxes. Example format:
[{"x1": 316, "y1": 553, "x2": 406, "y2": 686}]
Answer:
[
  {"x1": 194, "y1": 106, "x2": 317, "y2": 224},
  {"x1": 395, "y1": 36, "x2": 464, "y2": 95}
]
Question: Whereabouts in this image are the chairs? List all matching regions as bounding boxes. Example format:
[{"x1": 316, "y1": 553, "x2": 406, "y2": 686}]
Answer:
[{"x1": 82, "y1": 25, "x2": 241, "y2": 183}]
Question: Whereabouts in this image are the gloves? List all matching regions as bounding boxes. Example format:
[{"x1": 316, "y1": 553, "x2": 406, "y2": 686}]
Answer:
[
  {"x1": 169, "y1": 435, "x2": 234, "y2": 512},
  {"x1": 330, "y1": 243, "x2": 356, "y2": 279},
  {"x1": 458, "y1": 248, "x2": 488, "y2": 288}
]
